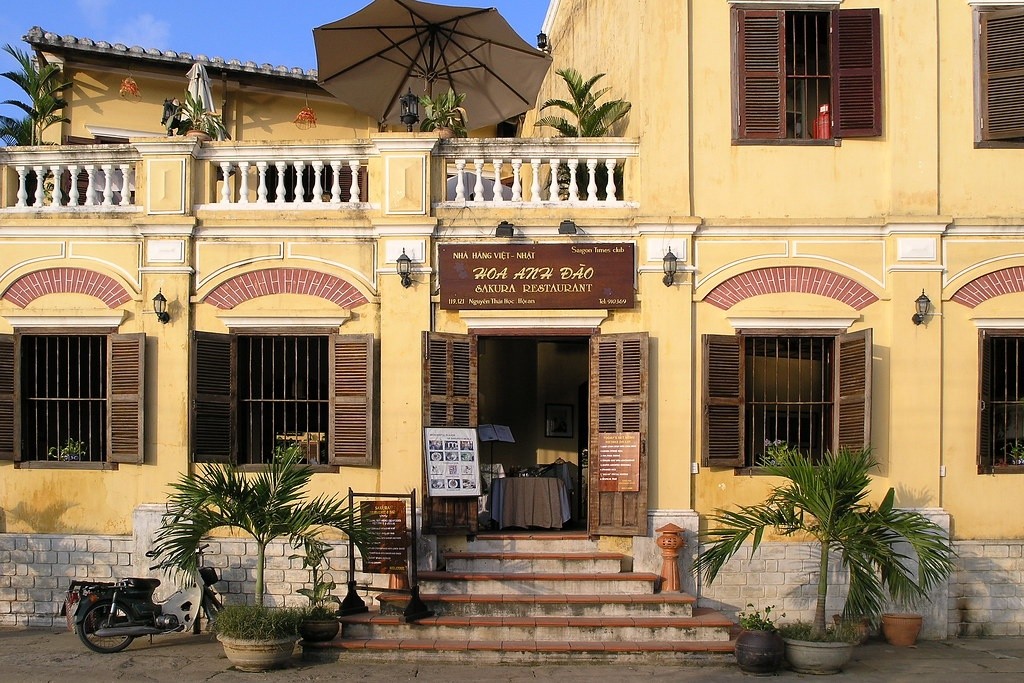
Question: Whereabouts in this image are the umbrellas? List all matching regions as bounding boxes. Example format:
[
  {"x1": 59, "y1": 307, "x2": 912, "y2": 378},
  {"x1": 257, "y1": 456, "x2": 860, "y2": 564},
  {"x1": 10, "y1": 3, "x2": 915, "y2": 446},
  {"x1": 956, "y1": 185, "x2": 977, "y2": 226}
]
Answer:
[
  {"x1": 181, "y1": 63, "x2": 225, "y2": 140},
  {"x1": 312, "y1": 0, "x2": 554, "y2": 130}
]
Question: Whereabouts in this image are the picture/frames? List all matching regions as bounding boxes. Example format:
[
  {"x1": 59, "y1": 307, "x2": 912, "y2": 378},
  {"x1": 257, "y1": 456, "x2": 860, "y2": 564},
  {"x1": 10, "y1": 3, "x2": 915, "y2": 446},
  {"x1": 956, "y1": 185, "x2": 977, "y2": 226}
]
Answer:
[{"x1": 544, "y1": 403, "x2": 574, "y2": 439}]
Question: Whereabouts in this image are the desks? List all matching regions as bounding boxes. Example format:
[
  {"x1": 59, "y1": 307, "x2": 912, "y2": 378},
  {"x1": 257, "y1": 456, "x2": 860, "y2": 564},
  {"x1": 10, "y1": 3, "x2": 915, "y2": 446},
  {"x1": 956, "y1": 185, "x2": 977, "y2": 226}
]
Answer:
[{"x1": 486, "y1": 476, "x2": 572, "y2": 531}]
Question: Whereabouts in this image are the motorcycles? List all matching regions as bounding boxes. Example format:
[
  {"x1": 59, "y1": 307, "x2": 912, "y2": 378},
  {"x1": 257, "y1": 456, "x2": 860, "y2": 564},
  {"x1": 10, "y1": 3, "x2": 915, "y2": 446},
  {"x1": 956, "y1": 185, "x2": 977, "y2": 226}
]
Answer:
[{"x1": 54, "y1": 543, "x2": 225, "y2": 653}]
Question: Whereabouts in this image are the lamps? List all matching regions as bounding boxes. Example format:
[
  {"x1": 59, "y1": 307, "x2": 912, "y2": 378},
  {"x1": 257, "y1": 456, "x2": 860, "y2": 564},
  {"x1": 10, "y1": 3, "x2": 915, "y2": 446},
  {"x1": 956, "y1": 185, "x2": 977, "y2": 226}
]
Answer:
[
  {"x1": 536, "y1": 31, "x2": 552, "y2": 54},
  {"x1": 399, "y1": 86, "x2": 421, "y2": 132},
  {"x1": 558, "y1": 220, "x2": 578, "y2": 234},
  {"x1": 161, "y1": 97, "x2": 183, "y2": 136},
  {"x1": 396, "y1": 247, "x2": 412, "y2": 289},
  {"x1": 662, "y1": 246, "x2": 677, "y2": 287},
  {"x1": 495, "y1": 221, "x2": 514, "y2": 237},
  {"x1": 912, "y1": 288, "x2": 930, "y2": 325},
  {"x1": 152, "y1": 287, "x2": 170, "y2": 324}
]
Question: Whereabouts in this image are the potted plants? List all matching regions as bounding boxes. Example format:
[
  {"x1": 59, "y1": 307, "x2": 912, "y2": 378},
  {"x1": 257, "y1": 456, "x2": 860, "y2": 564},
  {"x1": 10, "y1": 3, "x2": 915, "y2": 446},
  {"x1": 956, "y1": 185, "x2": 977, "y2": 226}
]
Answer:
[
  {"x1": 417, "y1": 86, "x2": 468, "y2": 138},
  {"x1": 152, "y1": 443, "x2": 382, "y2": 672},
  {"x1": 166, "y1": 89, "x2": 233, "y2": 141},
  {"x1": 687, "y1": 443, "x2": 962, "y2": 675},
  {"x1": 0, "y1": 42, "x2": 74, "y2": 206},
  {"x1": 48, "y1": 437, "x2": 87, "y2": 461}
]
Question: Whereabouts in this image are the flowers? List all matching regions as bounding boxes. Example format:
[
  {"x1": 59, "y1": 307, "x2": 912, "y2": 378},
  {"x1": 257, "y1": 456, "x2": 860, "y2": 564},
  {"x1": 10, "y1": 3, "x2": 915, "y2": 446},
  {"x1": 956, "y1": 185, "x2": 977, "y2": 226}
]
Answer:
[{"x1": 765, "y1": 438, "x2": 796, "y2": 465}]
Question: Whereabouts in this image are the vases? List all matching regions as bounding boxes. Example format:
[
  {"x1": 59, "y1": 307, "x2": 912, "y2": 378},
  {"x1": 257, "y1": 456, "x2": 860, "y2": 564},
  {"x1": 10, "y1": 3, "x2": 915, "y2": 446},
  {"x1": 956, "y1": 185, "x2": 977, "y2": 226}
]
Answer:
[
  {"x1": 832, "y1": 614, "x2": 871, "y2": 646},
  {"x1": 882, "y1": 614, "x2": 922, "y2": 647}
]
